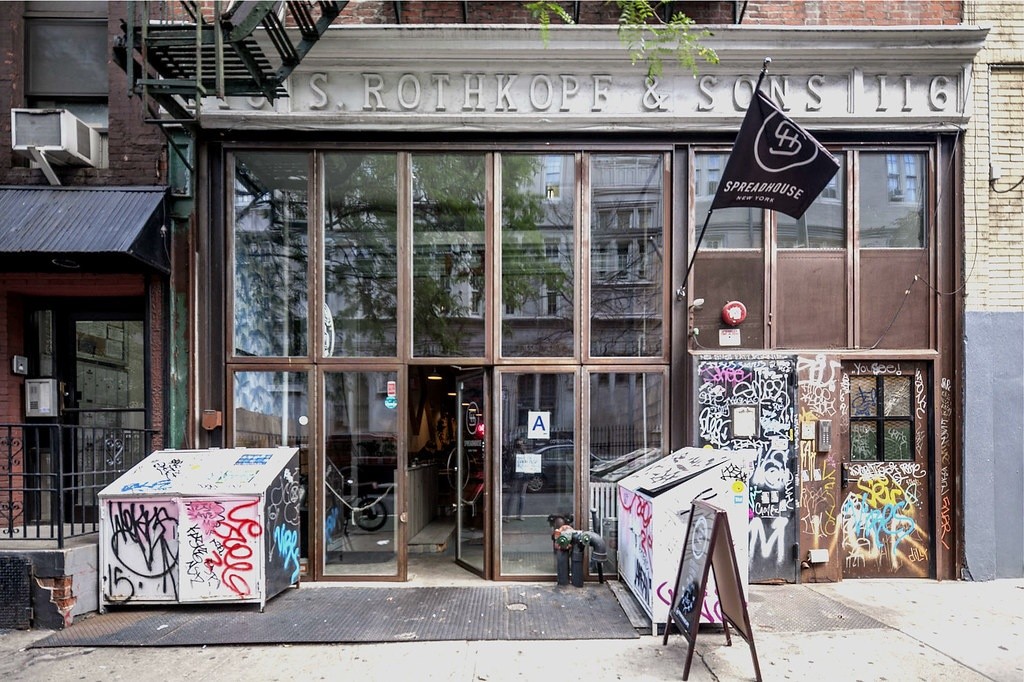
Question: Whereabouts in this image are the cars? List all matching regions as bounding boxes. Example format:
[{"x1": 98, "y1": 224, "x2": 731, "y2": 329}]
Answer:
[{"x1": 499, "y1": 433, "x2": 617, "y2": 495}]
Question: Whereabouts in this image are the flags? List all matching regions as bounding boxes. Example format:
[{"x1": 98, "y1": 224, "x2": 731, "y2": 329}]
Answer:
[{"x1": 711, "y1": 92, "x2": 841, "y2": 220}]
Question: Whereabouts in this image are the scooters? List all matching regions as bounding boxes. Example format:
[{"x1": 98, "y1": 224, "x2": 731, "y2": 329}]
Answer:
[{"x1": 324, "y1": 458, "x2": 388, "y2": 534}]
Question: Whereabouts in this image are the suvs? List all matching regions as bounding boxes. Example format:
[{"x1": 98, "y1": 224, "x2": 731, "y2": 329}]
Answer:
[{"x1": 322, "y1": 431, "x2": 398, "y2": 491}]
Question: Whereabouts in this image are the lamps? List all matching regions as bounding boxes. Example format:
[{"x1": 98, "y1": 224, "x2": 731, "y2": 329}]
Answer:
[{"x1": 427, "y1": 365, "x2": 442, "y2": 380}]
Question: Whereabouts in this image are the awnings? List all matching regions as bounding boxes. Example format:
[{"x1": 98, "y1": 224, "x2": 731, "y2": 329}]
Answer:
[{"x1": 1, "y1": 184, "x2": 171, "y2": 277}]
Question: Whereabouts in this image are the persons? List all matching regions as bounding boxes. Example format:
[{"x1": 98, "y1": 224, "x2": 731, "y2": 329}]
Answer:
[
  {"x1": 324, "y1": 419, "x2": 349, "y2": 541},
  {"x1": 502, "y1": 438, "x2": 531, "y2": 523}
]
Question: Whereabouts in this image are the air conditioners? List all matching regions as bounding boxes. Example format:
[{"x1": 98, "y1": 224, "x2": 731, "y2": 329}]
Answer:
[{"x1": 11, "y1": 107, "x2": 100, "y2": 166}]
